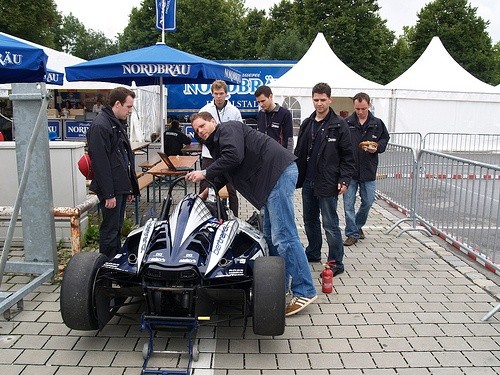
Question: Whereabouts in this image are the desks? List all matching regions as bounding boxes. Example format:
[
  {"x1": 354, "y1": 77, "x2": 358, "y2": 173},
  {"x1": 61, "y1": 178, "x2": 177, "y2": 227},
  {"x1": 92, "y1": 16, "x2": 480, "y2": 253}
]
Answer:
[
  {"x1": 181, "y1": 143, "x2": 202, "y2": 156},
  {"x1": 131, "y1": 142, "x2": 151, "y2": 160},
  {"x1": 147, "y1": 156, "x2": 199, "y2": 203}
]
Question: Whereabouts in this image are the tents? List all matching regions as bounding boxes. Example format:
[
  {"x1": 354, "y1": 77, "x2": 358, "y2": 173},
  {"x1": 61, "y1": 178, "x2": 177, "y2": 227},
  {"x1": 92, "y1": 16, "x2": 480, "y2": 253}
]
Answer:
[
  {"x1": 374, "y1": 36, "x2": 500, "y2": 153},
  {"x1": 257, "y1": 32, "x2": 393, "y2": 149}
]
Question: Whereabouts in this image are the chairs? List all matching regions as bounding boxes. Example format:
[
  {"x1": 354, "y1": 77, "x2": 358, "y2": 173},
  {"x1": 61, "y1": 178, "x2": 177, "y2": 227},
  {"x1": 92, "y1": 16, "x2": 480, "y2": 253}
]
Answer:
[{"x1": 160, "y1": 175, "x2": 221, "y2": 221}]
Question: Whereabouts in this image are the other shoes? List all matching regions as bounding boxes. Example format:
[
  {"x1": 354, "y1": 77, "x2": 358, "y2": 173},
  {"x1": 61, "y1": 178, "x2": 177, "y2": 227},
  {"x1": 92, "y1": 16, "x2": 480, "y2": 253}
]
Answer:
[
  {"x1": 344, "y1": 237, "x2": 358, "y2": 246},
  {"x1": 358, "y1": 228, "x2": 364, "y2": 239},
  {"x1": 306, "y1": 257, "x2": 321, "y2": 263},
  {"x1": 320, "y1": 266, "x2": 344, "y2": 278}
]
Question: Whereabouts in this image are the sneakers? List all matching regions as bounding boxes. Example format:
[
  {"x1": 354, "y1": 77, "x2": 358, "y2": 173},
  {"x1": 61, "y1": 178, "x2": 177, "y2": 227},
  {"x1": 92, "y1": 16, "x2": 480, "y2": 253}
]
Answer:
[{"x1": 285, "y1": 292, "x2": 318, "y2": 317}]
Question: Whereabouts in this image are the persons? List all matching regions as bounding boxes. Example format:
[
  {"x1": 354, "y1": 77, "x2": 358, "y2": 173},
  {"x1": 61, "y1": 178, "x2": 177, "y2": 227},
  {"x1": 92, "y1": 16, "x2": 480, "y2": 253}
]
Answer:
[
  {"x1": 164, "y1": 119, "x2": 192, "y2": 157},
  {"x1": 251, "y1": 86, "x2": 294, "y2": 149},
  {"x1": 0, "y1": 108, "x2": 13, "y2": 141},
  {"x1": 87, "y1": 87, "x2": 137, "y2": 260},
  {"x1": 63, "y1": 99, "x2": 106, "y2": 113},
  {"x1": 196, "y1": 80, "x2": 240, "y2": 219},
  {"x1": 343, "y1": 92, "x2": 390, "y2": 246},
  {"x1": 294, "y1": 82, "x2": 357, "y2": 277},
  {"x1": 184, "y1": 112, "x2": 318, "y2": 316}
]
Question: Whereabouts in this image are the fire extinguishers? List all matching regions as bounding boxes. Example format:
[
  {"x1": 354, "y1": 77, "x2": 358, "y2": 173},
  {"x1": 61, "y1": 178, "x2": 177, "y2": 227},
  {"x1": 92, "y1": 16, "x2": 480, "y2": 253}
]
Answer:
[{"x1": 322, "y1": 260, "x2": 337, "y2": 293}]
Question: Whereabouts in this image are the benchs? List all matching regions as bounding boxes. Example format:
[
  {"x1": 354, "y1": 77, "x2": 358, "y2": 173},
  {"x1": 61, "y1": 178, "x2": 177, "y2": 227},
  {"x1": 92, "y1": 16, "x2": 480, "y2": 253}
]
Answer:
[{"x1": 89, "y1": 157, "x2": 161, "y2": 226}]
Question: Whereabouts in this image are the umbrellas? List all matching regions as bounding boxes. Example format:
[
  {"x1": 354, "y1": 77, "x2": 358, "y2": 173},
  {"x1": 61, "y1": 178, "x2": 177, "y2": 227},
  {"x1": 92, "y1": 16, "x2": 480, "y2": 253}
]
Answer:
[
  {"x1": 65, "y1": 43, "x2": 243, "y2": 154},
  {"x1": 0, "y1": 34, "x2": 49, "y2": 85}
]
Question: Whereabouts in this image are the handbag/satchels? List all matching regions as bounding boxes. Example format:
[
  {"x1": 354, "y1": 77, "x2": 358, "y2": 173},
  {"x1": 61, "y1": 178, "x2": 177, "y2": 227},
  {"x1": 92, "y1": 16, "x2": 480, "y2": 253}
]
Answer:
[{"x1": 77, "y1": 152, "x2": 94, "y2": 181}]
